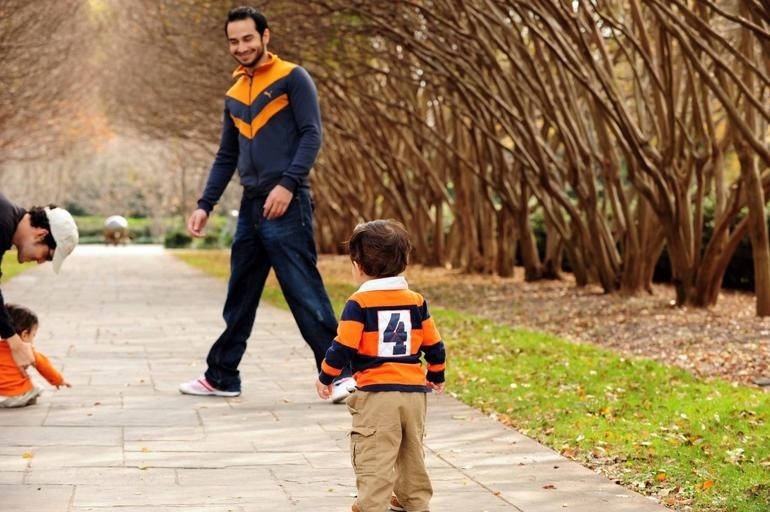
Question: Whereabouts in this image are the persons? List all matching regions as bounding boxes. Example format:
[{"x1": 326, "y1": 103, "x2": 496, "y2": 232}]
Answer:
[
  {"x1": 0, "y1": 303, "x2": 72, "y2": 408},
  {"x1": 317, "y1": 217, "x2": 445, "y2": 512},
  {"x1": 179, "y1": 5, "x2": 356, "y2": 404},
  {"x1": 1, "y1": 188, "x2": 79, "y2": 380}
]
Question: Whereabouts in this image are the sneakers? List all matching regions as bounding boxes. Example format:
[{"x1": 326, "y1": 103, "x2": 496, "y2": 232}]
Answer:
[
  {"x1": 331, "y1": 377, "x2": 357, "y2": 403},
  {"x1": 352, "y1": 496, "x2": 405, "y2": 512},
  {"x1": 180, "y1": 376, "x2": 241, "y2": 397}
]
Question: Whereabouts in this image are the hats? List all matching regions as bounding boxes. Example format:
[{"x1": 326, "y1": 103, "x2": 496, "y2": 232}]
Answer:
[{"x1": 44, "y1": 206, "x2": 79, "y2": 274}]
